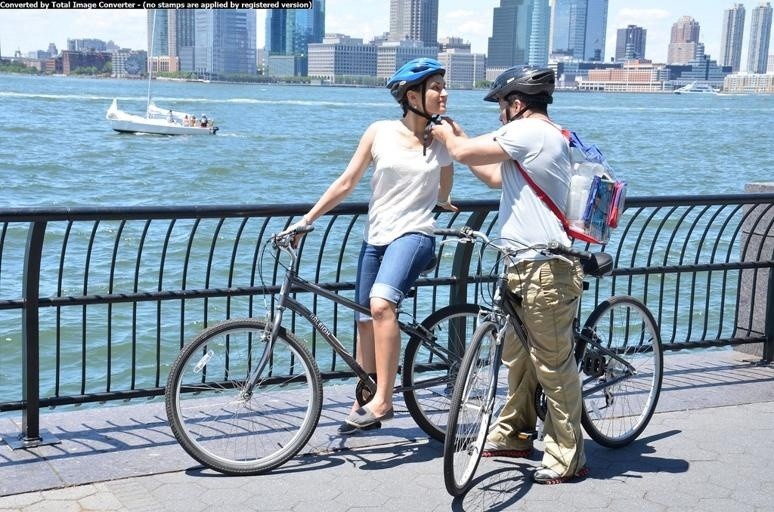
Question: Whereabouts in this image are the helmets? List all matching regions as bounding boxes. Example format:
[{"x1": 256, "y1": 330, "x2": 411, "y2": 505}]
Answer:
[
  {"x1": 386, "y1": 58, "x2": 445, "y2": 103},
  {"x1": 484, "y1": 64, "x2": 555, "y2": 104}
]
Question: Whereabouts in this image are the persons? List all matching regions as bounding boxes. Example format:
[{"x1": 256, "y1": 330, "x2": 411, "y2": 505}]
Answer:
[
  {"x1": 190, "y1": 113, "x2": 197, "y2": 127},
  {"x1": 166, "y1": 109, "x2": 174, "y2": 123},
  {"x1": 277, "y1": 56, "x2": 461, "y2": 438},
  {"x1": 182, "y1": 114, "x2": 189, "y2": 127},
  {"x1": 428, "y1": 62, "x2": 592, "y2": 485},
  {"x1": 199, "y1": 113, "x2": 208, "y2": 128}
]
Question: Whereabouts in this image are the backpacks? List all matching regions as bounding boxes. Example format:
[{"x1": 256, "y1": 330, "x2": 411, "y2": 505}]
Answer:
[{"x1": 513, "y1": 120, "x2": 626, "y2": 244}]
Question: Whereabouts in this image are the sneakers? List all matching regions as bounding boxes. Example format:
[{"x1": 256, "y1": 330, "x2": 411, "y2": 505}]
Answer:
[
  {"x1": 467, "y1": 439, "x2": 533, "y2": 457},
  {"x1": 337, "y1": 406, "x2": 393, "y2": 434},
  {"x1": 529, "y1": 465, "x2": 589, "y2": 484}
]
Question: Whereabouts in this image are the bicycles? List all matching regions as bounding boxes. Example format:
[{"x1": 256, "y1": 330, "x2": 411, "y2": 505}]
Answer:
[
  {"x1": 431, "y1": 223, "x2": 664, "y2": 498},
  {"x1": 163, "y1": 224, "x2": 501, "y2": 479}
]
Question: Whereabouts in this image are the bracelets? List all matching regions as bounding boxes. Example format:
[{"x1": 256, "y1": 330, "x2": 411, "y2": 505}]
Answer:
[
  {"x1": 303, "y1": 214, "x2": 313, "y2": 225},
  {"x1": 435, "y1": 195, "x2": 454, "y2": 208}
]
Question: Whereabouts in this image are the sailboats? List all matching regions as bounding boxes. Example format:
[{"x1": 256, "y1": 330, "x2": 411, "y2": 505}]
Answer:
[{"x1": 106, "y1": 10, "x2": 219, "y2": 136}]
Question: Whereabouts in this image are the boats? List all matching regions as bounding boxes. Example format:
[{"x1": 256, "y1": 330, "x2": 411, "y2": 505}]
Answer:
[{"x1": 672, "y1": 81, "x2": 720, "y2": 96}]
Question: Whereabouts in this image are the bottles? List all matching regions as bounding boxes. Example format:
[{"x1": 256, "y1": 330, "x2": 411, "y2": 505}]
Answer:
[{"x1": 572, "y1": 162, "x2": 604, "y2": 180}]
